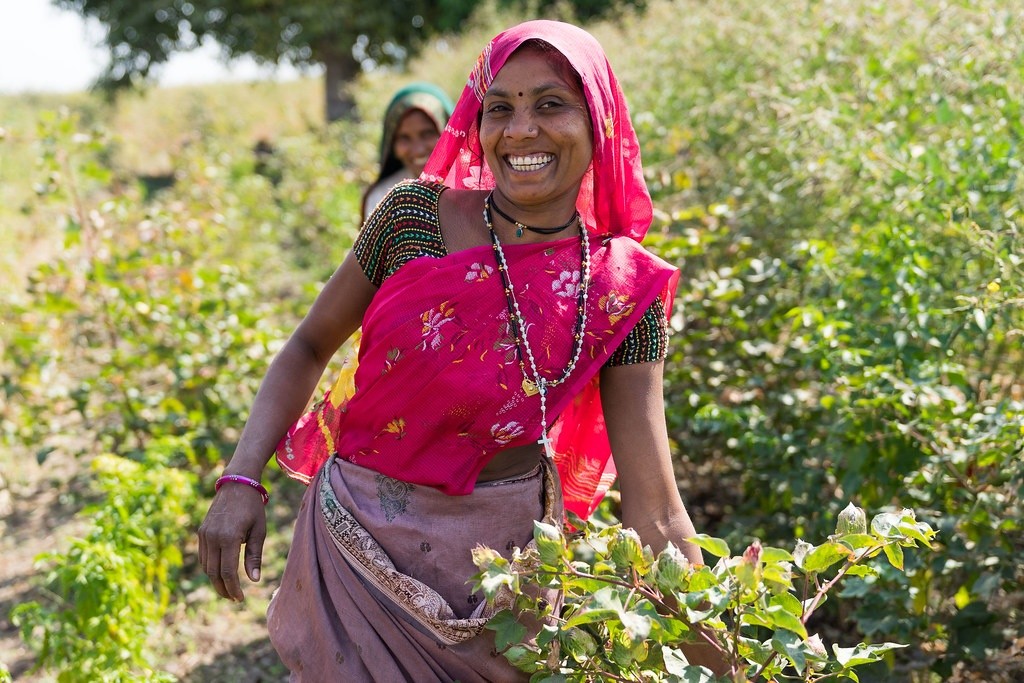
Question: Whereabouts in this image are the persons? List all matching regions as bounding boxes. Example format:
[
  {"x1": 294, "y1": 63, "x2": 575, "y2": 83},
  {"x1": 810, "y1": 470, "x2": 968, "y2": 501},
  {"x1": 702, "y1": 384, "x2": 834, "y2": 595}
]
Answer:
[
  {"x1": 361, "y1": 82, "x2": 456, "y2": 227},
  {"x1": 196, "y1": 19, "x2": 736, "y2": 683}
]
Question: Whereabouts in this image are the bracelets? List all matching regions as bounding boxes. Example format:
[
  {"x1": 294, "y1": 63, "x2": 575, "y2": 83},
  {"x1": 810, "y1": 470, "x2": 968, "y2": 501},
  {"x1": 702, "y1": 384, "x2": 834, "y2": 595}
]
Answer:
[{"x1": 215, "y1": 474, "x2": 270, "y2": 505}]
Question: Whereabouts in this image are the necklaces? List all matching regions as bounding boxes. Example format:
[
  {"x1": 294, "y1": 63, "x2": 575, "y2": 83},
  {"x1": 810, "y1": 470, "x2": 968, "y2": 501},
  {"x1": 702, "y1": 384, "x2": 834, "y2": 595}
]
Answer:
[{"x1": 482, "y1": 190, "x2": 591, "y2": 459}]
